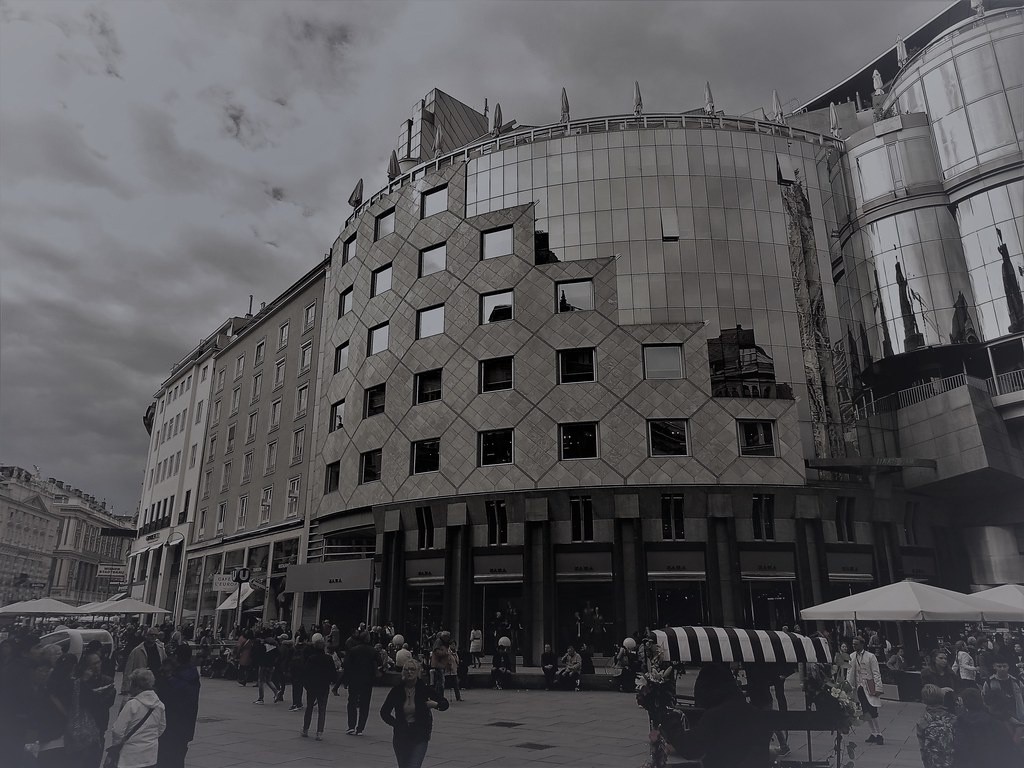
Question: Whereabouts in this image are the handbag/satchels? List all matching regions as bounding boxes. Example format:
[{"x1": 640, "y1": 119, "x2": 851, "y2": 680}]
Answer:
[
  {"x1": 103, "y1": 744, "x2": 121, "y2": 768},
  {"x1": 866, "y1": 679, "x2": 884, "y2": 696}
]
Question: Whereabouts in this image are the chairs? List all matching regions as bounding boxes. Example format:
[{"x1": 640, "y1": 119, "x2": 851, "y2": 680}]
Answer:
[{"x1": 878, "y1": 663, "x2": 904, "y2": 702}]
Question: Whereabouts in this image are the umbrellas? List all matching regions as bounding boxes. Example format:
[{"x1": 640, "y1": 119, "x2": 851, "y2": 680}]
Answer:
[
  {"x1": 896, "y1": 34, "x2": 908, "y2": 68},
  {"x1": 829, "y1": 102, "x2": 842, "y2": 136},
  {"x1": 387, "y1": 150, "x2": 401, "y2": 178},
  {"x1": 873, "y1": 70, "x2": 885, "y2": 96},
  {"x1": 772, "y1": 90, "x2": 783, "y2": 119},
  {"x1": 703, "y1": 80, "x2": 714, "y2": 115},
  {"x1": 0, "y1": 597, "x2": 172, "y2": 628},
  {"x1": 491, "y1": 103, "x2": 502, "y2": 139},
  {"x1": 560, "y1": 87, "x2": 570, "y2": 124},
  {"x1": 799, "y1": 580, "x2": 1024, "y2": 622},
  {"x1": 633, "y1": 81, "x2": 643, "y2": 112},
  {"x1": 348, "y1": 178, "x2": 363, "y2": 207},
  {"x1": 432, "y1": 125, "x2": 442, "y2": 153}
]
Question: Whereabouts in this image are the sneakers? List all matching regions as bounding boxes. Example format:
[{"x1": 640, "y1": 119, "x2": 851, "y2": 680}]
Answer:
[{"x1": 777, "y1": 746, "x2": 791, "y2": 757}]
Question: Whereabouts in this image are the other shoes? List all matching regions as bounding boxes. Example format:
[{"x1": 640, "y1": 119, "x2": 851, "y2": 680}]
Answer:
[
  {"x1": 876, "y1": 735, "x2": 883, "y2": 745},
  {"x1": 865, "y1": 734, "x2": 877, "y2": 742},
  {"x1": 346, "y1": 729, "x2": 354, "y2": 734},
  {"x1": 253, "y1": 699, "x2": 264, "y2": 705},
  {"x1": 302, "y1": 729, "x2": 308, "y2": 736},
  {"x1": 289, "y1": 705, "x2": 301, "y2": 711},
  {"x1": 497, "y1": 684, "x2": 502, "y2": 690},
  {"x1": 315, "y1": 732, "x2": 322, "y2": 741},
  {"x1": 479, "y1": 662, "x2": 482, "y2": 667},
  {"x1": 274, "y1": 690, "x2": 281, "y2": 703},
  {"x1": 239, "y1": 681, "x2": 246, "y2": 686},
  {"x1": 253, "y1": 684, "x2": 258, "y2": 687},
  {"x1": 356, "y1": 730, "x2": 362, "y2": 735},
  {"x1": 457, "y1": 697, "x2": 464, "y2": 701},
  {"x1": 332, "y1": 689, "x2": 339, "y2": 696},
  {"x1": 472, "y1": 665, "x2": 476, "y2": 668}
]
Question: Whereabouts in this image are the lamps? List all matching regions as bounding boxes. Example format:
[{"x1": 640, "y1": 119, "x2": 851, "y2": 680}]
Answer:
[{"x1": 164, "y1": 531, "x2": 185, "y2": 548}]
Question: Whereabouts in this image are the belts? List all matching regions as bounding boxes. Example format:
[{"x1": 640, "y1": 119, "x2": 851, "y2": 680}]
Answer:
[{"x1": 474, "y1": 637, "x2": 480, "y2": 640}]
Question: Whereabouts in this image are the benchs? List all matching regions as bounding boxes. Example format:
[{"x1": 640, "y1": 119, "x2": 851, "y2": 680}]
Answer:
[{"x1": 664, "y1": 703, "x2": 849, "y2": 768}]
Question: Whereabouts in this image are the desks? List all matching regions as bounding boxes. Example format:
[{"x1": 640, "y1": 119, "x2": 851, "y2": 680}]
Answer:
[{"x1": 903, "y1": 670, "x2": 922, "y2": 702}]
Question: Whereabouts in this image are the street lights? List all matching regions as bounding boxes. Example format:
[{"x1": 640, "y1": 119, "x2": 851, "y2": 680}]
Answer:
[{"x1": 164, "y1": 532, "x2": 185, "y2": 627}]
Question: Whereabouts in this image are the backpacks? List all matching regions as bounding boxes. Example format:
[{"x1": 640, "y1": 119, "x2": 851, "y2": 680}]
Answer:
[{"x1": 922, "y1": 712, "x2": 956, "y2": 767}]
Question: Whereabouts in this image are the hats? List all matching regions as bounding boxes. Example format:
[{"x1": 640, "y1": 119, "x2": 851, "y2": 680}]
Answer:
[{"x1": 277, "y1": 634, "x2": 288, "y2": 640}]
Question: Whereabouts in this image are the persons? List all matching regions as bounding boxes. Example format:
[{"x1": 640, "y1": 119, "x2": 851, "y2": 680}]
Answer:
[
  {"x1": 332, "y1": 632, "x2": 383, "y2": 735},
  {"x1": 541, "y1": 643, "x2": 595, "y2": 691},
  {"x1": 0, "y1": 627, "x2": 201, "y2": 768},
  {"x1": 584, "y1": 601, "x2": 607, "y2": 634},
  {"x1": 493, "y1": 602, "x2": 523, "y2": 650},
  {"x1": 798, "y1": 626, "x2": 1024, "y2": 768},
  {"x1": 490, "y1": 646, "x2": 512, "y2": 690},
  {"x1": 93, "y1": 616, "x2": 413, "y2": 711},
  {"x1": 380, "y1": 659, "x2": 449, "y2": 768},
  {"x1": 469, "y1": 623, "x2": 483, "y2": 668},
  {"x1": 301, "y1": 639, "x2": 338, "y2": 741},
  {"x1": 662, "y1": 658, "x2": 796, "y2": 768},
  {"x1": 430, "y1": 631, "x2": 465, "y2": 701}
]
